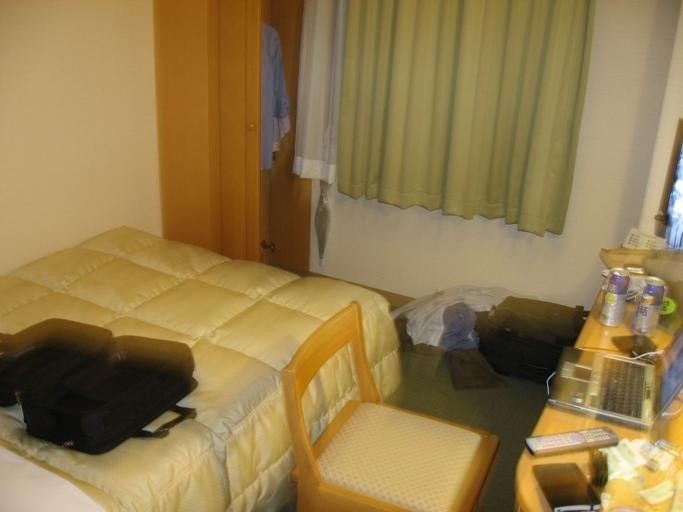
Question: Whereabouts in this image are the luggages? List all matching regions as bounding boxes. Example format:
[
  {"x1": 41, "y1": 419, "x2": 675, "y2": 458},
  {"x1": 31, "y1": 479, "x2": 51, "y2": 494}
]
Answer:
[{"x1": 477, "y1": 296, "x2": 589, "y2": 384}]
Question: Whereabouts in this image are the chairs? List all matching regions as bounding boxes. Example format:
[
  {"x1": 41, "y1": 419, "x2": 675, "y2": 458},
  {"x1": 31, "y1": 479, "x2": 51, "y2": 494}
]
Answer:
[{"x1": 279, "y1": 300, "x2": 500, "y2": 511}]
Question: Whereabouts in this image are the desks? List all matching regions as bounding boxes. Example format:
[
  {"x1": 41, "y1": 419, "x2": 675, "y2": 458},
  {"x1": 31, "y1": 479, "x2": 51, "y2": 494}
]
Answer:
[{"x1": 515, "y1": 239, "x2": 682, "y2": 512}]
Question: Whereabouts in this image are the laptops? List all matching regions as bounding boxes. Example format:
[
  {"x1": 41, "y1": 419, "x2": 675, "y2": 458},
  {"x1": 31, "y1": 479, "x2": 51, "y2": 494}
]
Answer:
[{"x1": 547, "y1": 321, "x2": 683, "y2": 431}]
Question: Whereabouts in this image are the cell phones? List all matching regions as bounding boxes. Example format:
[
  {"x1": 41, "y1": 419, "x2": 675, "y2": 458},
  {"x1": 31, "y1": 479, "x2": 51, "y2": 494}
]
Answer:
[{"x1": 612, "y1": 334, "x2": 656, "y2": 355}]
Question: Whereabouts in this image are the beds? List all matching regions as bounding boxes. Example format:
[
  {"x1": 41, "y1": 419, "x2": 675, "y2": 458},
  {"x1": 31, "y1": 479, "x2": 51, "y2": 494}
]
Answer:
[{"x1": 1, "y1": 224, "x2": 400, "y2": 511}]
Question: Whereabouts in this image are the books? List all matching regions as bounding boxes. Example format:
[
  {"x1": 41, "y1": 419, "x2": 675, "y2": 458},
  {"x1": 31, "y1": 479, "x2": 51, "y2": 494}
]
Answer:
[{"x1": 531, "y1": 462, "x2": 603, "y2": 512}]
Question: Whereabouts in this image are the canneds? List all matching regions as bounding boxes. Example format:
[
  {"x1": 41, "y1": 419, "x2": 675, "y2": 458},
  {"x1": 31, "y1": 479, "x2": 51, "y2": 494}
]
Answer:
[
  {"x1": 633, "y1": 277, "x2": 667, "y2": 333},
  {"x1": 596, "y1": 267, "x2": 629, "y2": 328}
]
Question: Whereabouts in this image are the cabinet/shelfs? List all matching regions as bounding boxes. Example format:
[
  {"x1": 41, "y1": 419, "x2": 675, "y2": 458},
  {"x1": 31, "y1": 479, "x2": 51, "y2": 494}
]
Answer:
[{"x1": 152, "y1": 0, "x2": 313, "y2": 280}]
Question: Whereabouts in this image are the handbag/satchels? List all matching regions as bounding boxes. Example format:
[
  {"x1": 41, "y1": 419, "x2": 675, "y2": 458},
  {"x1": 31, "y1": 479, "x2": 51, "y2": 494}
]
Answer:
[{"x1": 0, "y1": 318, "x2": 199, "y2": 454}]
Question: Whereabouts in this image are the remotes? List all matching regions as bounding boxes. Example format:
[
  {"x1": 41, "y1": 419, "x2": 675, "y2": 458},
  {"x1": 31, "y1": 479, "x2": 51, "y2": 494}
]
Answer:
[{"x1": 524, "y1": 426, "x2": 618, "y2": 456}]
